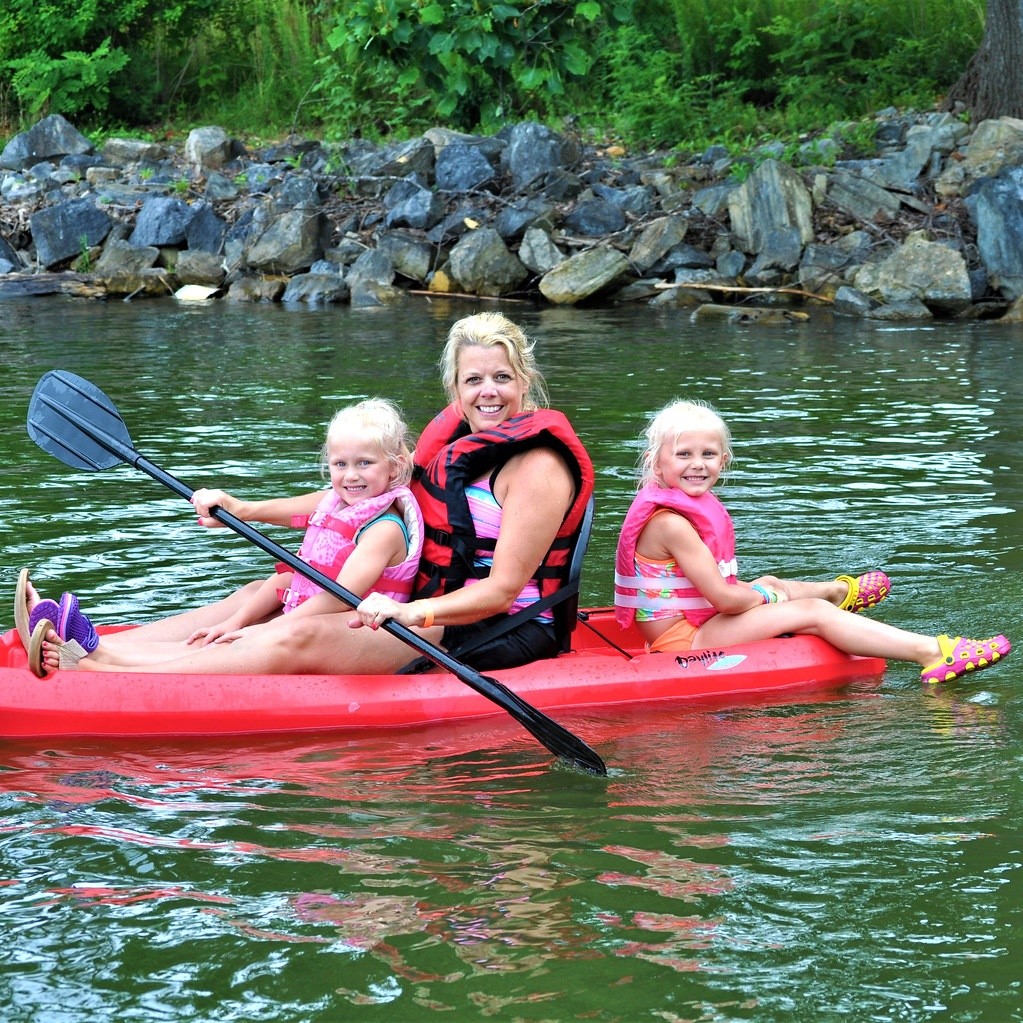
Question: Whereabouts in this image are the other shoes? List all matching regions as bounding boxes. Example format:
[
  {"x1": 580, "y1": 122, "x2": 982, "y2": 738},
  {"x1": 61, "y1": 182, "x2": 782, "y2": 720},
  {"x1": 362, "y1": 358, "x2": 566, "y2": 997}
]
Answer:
[{"x1": 30, "y1": 591, "x2": 99, "y2": 654}]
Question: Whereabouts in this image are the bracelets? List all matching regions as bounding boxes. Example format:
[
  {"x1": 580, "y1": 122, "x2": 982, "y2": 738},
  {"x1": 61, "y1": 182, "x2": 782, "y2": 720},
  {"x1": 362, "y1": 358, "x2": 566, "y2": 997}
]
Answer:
[
  {"x1": 752, "y1": 586, "x2": 780, "y2": 604},
  {"x1": 415, "y1": 599, "x2": 434, "y2": 628}
]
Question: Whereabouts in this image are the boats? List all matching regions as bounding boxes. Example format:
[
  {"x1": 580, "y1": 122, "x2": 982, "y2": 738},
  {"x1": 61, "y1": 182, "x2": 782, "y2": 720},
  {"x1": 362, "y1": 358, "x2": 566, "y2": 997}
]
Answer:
[{"x1": 0, "y1": 621, "x2": 888, "y2": 738}]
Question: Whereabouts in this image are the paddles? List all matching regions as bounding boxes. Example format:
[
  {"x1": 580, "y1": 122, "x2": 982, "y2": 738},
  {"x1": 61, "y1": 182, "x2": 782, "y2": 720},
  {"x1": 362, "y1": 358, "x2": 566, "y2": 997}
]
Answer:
[{"x1": 26, "y1": 368, "x2": 612, "y2": 780}]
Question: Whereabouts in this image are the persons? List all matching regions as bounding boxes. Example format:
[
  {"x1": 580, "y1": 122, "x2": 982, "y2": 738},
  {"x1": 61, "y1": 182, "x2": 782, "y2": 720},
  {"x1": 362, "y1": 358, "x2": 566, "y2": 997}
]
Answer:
[
  {"x1": 616, "y1": 402, "x2": 1011, "y2": 685},
  {"x1": 15, "y1": 306, "x2": 600, "y2": 674},
  {"x1": 29, "y1": 396, "x2": 420, "y2": 666}
]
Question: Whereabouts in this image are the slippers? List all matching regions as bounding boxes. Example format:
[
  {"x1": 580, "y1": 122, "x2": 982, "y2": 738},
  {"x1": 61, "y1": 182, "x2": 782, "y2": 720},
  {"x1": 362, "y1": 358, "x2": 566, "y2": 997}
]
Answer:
[
  {"x1": 29, "y1": 618, "x2": 89, "y2": 680},
  {"x1": 920, "y1": 633, "x2": 1011, "y2": 684},
  {"x1": 835, "y1": 571, "x2": 891, "y2": 613},
  {"x1": 15, "y1": 569, "x2": 31, "y2": 656}
]
